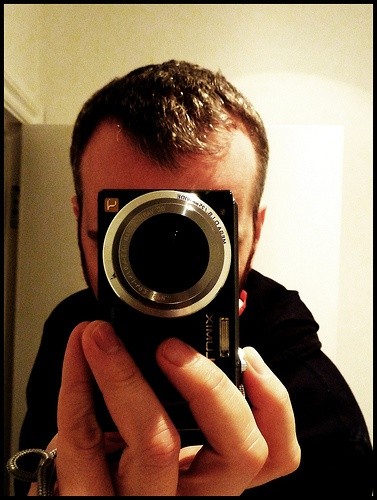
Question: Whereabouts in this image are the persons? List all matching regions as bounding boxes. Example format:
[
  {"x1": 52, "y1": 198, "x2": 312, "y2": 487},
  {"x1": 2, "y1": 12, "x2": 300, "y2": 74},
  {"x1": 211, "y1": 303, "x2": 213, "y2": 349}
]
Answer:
[{"x1": 12, "y1": 60, "x2": 373, "y2": 500}]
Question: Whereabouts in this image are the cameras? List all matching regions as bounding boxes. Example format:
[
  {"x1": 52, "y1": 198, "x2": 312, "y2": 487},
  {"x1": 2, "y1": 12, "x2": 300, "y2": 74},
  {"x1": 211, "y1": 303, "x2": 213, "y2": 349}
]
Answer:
[{"x1": 90, "y1": 188, "x2": 249, "y2": 445}]
